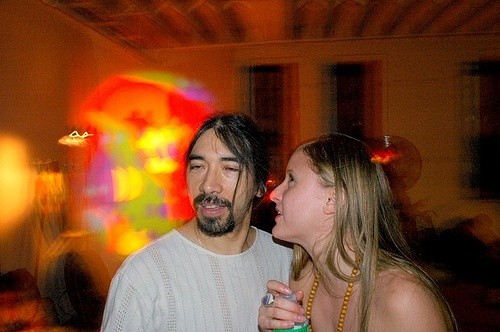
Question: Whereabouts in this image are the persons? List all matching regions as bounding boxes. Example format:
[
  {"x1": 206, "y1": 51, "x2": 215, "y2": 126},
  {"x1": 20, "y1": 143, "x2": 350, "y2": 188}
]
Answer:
[
  {"x1": 101, "y1": 112, "x2": 295, "y2": 332},
  {"x1": 257, "y1": 133, "x2": 458, "y2": 332}
]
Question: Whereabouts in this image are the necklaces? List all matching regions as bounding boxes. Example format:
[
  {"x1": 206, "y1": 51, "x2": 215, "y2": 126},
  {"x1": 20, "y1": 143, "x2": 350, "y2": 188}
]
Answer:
[
  {"x1": 192, "y1": 220, "x2": 204, "y2": 248},
  {"x1": 305, "y1": 252, "x2": 364, "y2": 332}
]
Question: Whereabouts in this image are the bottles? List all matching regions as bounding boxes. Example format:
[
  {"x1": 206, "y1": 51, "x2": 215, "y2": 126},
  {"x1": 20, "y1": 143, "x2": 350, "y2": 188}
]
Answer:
[{"x1": 271, "y1": 291, "x2": 309, "y2": 332}]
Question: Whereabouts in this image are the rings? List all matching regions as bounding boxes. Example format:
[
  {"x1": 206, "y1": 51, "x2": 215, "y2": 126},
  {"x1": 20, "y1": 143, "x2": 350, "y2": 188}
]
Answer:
[{"x1": 262, "y1": 293, "x2": 275, "y2": 307}]
